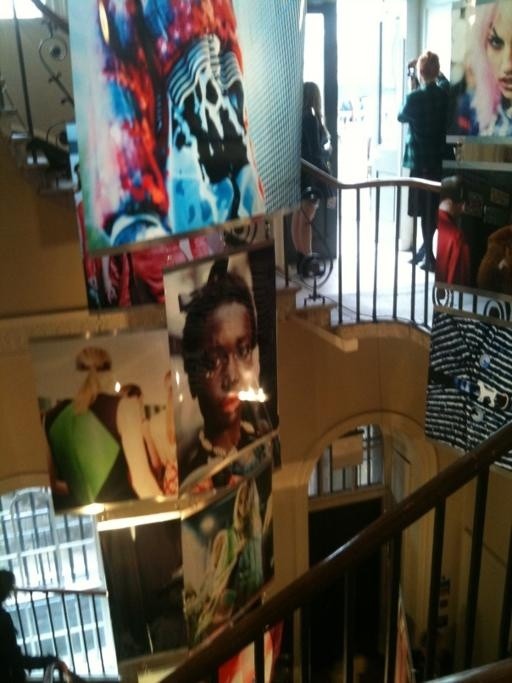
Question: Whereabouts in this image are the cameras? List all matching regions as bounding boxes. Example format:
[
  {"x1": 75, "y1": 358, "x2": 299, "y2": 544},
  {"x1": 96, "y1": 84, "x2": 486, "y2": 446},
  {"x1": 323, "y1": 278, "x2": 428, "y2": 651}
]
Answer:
[{"x1": 407, "y1": 67, "x2": 417, "y2": 79}]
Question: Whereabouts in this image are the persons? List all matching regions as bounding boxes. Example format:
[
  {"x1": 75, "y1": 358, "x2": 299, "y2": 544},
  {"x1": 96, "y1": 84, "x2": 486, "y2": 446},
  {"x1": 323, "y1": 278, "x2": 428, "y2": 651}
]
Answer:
[
  {"x1": 435, "y1": 176, "x2": 475, "y2": 284},
  {"x1": 289, "y1": 81, "x2": 331, "y2": 278},
  {"x1": 0, "y1": 569, "x2": 68, "y2": 683},
  {"x1": 180, "y1": 270, "x2": 259, "y2": 486},
  {"x1": 45, "y1": 347, "x2": 179, "y2": 513},
  {"x1": 398, "y1": 49, "x2": 458, "y2": 271},
  {"x1": 189, "y1": 479, "x2": 263, "y2": 645},
  {"x1": 450, "y1": 0, "x2": 512, "y2": 138}
]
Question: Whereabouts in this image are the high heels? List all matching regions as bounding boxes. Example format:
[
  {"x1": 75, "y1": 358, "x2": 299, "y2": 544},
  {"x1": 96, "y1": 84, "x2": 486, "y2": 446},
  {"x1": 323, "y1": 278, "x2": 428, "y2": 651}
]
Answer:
[
  {"x1": 407, "y1": 254, "x2": 435, "y2": 272},
  {"x1": 296, "y1": 251, "x2": 326, "y2": 278}
]
